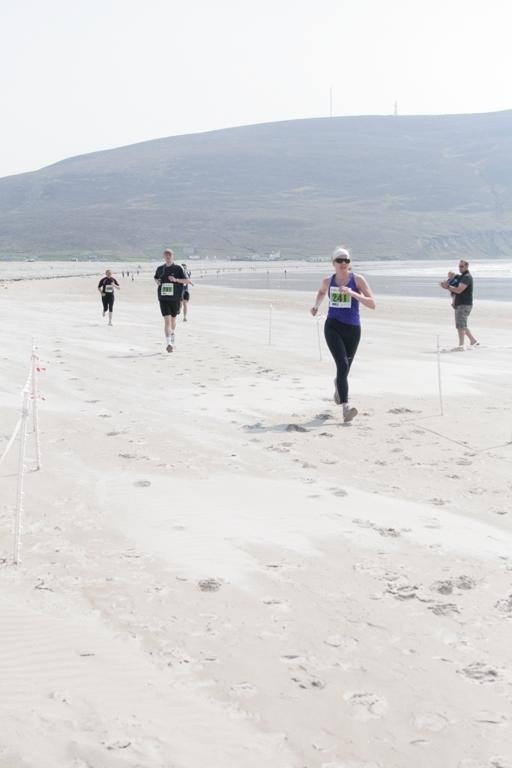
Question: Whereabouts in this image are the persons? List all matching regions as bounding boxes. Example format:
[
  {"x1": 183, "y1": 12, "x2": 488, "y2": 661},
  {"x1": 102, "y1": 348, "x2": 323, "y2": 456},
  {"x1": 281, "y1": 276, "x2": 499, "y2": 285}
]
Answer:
[
  {"x1": 310, "y1": 247, "x2": 376, "y2": 423},
  {"x1": 121, "y1": 264, "x2": 287, "y2": 282},
  {"x1": 179, "y1": 263, "x2": 196, "y2": 323},
  {"x1": 153, "y1": 249, "x2": 190, "y2": 353},
  {"x1": 441, "y1": 259, "x2": 481, "y2": 351},
  {"x1": 441, "y1": 270, "x2": 460, "y2": 311},
  {"x1": 97, "y1": 267, "x2": 119, "y2": 328}
]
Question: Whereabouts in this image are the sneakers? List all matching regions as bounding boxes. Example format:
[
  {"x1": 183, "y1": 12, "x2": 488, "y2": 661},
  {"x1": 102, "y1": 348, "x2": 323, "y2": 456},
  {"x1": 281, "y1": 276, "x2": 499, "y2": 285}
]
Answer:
[
  {"x1": 167, "y1": 345, "x2": 173, "y2": 352},
  {"x1": 343, "y1": 408, "x2": 357, "y2": 422},
  {"x1": 468, "y1": 340, "x2": 479, "y2": 350},
  {"x1": 171, "y1": 334, "x2": 174, "y2": 342},
  {"x1": 451, "y1": 346, "x2": 464, "y2": 351},
  {"x1": 334, "y1": 378, "x2": 342, "y2": 404}
]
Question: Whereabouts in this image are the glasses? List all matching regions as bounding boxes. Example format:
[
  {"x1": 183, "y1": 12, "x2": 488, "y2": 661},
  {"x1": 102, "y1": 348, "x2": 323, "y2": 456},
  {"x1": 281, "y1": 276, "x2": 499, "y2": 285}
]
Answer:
[{"x1": 335, "y1": 258, "x2": 350, "y2": 263}]
